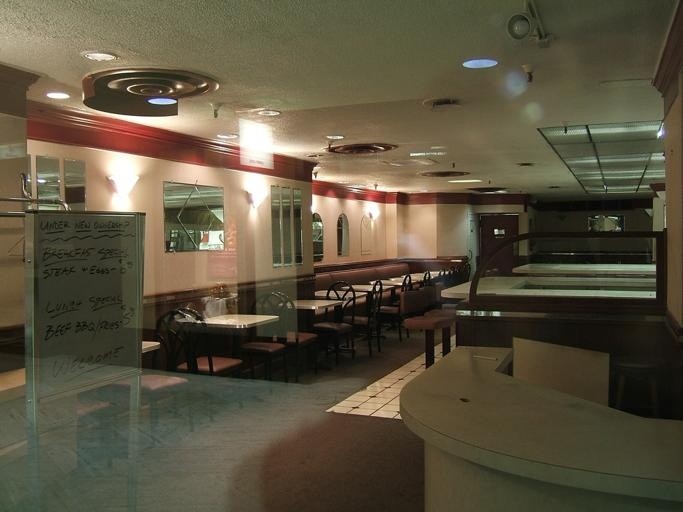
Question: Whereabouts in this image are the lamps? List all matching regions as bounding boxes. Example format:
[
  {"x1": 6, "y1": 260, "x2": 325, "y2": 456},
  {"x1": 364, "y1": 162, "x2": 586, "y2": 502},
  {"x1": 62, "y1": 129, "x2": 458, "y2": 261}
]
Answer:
[{"x1": 504, "y1": 0, "x2": 538, "y2": 43}]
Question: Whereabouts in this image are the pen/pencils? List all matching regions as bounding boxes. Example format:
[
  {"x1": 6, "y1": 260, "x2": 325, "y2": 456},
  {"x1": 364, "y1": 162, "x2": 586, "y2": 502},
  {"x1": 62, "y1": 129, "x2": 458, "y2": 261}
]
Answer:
[{"x1": 471, "y1": 356, "x2": 497, "y2": 361}]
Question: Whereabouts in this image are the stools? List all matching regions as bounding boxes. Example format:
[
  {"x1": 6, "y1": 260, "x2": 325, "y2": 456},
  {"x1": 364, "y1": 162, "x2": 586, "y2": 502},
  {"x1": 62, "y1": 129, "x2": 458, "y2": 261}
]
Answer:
[
  {"x1": 398, "y1": 288, "x2": 451, "y2": 370},
  {"x1": 420, "y1": 285, "x2": 458, "y2": 355},
  {"x1": 612, "y1": 351, "x2": 666, "y2": 416}
]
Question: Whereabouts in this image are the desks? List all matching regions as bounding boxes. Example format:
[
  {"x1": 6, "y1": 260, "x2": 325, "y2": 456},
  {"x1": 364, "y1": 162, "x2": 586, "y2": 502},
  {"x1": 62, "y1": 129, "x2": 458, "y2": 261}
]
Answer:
[
  {"x1": 179, "y1": 312, "x2": 278, "y2": 358},
  {"x1": 0, "y1": 336, "x2": 161, "y2": 404},
  {"x1": 279, "y1": 298, "x2": 343, "y2": 332}
]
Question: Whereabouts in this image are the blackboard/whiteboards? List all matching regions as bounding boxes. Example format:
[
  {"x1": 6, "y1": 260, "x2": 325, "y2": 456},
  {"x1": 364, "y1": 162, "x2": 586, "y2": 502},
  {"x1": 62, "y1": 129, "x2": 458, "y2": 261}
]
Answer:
[{"x1": 25, "y1": 210, "x2": 146, "y2": 404}]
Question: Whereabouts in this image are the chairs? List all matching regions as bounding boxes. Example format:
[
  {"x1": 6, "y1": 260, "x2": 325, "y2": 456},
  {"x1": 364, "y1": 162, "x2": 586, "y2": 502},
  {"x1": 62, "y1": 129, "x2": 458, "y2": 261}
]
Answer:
[
  {"x1": 263, "y1": 292, "x2": 319, "y2": 382},
  {"x1": 242, "y1": 293, "x2": 289, "y2": 382},
  {"x1": 314, "y1": 256, "x2": 471, "y2": 312},
  {"x1": 156, "y1": 308, "x2": 241, "y2": 378},
  {"x1": 343, "y1": 280, "x2": 384, "y2": 356},
  {"x1": 511, "y1": 335, "x2": 610, "y2": 405},
  {"x1": 313, "y1": 281, "x2": 355, "y2": 364}
]
